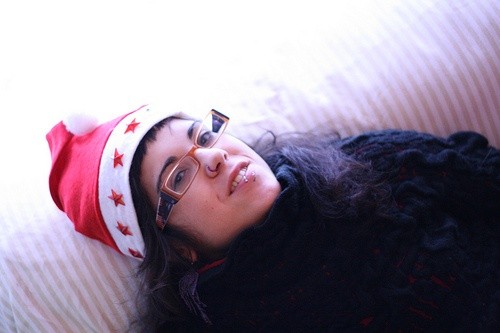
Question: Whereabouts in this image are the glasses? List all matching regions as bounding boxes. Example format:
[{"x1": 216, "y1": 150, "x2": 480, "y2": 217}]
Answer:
[{"x1": 157, "y1": 110, "x2": 230, "y2": 233}]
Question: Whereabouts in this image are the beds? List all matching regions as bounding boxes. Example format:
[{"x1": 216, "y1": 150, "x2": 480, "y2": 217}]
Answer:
[{"x1": 2, "y1": 0, "x2": 500, "y2": 333}]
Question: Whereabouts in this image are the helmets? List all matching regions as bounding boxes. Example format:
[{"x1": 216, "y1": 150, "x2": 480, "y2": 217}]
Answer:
[{"x1": 41, "y1": 104, "x2": 197, "y2": 261}]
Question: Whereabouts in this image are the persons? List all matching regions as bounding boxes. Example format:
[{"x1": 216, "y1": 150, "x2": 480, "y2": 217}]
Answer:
[{"x1": 48, "y1": 98, "x2": 500, "y2": 333}]
